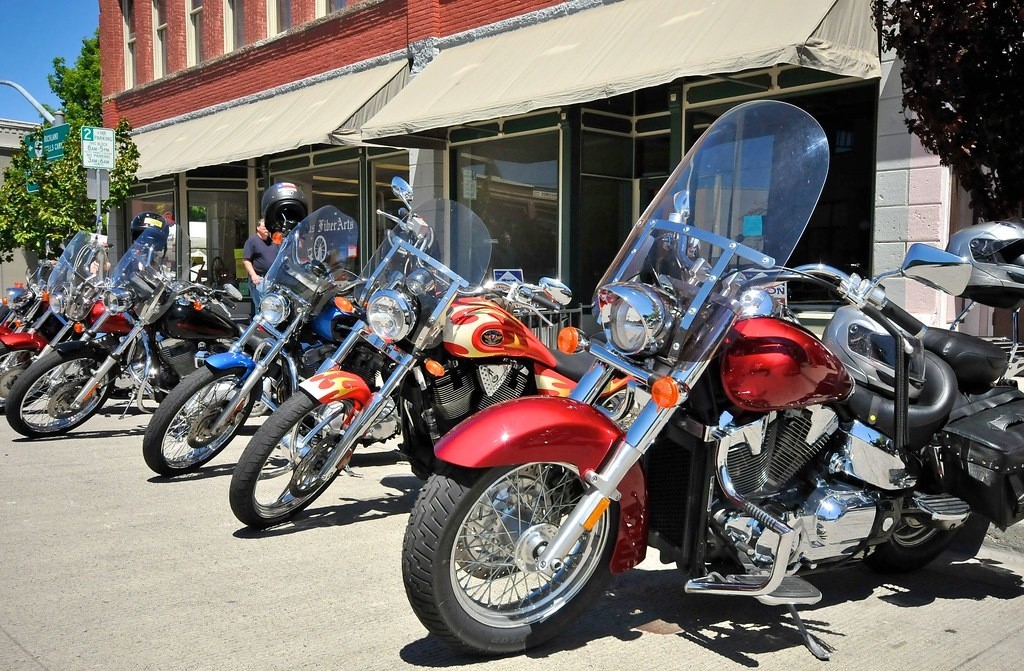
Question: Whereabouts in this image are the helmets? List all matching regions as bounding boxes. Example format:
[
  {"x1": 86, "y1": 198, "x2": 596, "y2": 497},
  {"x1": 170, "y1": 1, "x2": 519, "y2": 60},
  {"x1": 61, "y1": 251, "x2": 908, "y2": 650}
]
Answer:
[
  {"x1": 131, "y1": 212, "x2": 169, "y2": 250},
  {"x1": 260, "y1": 181, "x2": 309, "y2": 233},
  {"x1": 821, "y1": 305, "x2": 928, "y2": 404},
  {"x1": 37, "y1": 260, "x2": 67, "y2": 294},
  {"x1": 383, "y1": 227, "x2": 442, "y2": 283},
  {"x1": 941, "y1": 221, "x2": 1024, "y2": 308},
  {"x1": 6, "y1": 288, "x2": 24, "y2": 304}
]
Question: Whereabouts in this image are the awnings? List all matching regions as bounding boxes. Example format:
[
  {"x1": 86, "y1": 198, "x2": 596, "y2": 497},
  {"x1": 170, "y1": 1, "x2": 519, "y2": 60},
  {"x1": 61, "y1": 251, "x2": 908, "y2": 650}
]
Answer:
[
  {"x1": 107, "y1": 59, "x2": 411, "y2": 180},
  {"x1": 360, "y1": 0, "x2": 883, "y2": 150}
]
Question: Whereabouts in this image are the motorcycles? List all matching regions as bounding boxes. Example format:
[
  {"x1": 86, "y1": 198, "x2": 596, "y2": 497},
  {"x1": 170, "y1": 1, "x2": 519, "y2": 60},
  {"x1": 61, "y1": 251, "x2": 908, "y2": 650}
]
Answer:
[
  {"x1": 140, "y1": 183, "x2": 403, "y2": 481},
  {"x1": 402, "y1": 98, "x2": 1023, "y2": 662},
  {"x1": 226, "y1": 179, "x2": 610, "y2": 533},
  {"x1": 0, "y1": 212, "x2": 283, "y2": 442}
]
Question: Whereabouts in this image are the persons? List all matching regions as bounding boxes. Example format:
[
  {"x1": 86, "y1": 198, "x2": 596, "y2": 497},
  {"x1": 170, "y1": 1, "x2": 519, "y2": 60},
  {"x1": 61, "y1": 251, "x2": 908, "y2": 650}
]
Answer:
[{"x1": 242, "y1": 218, "x2": 278, "y2": 315}]
